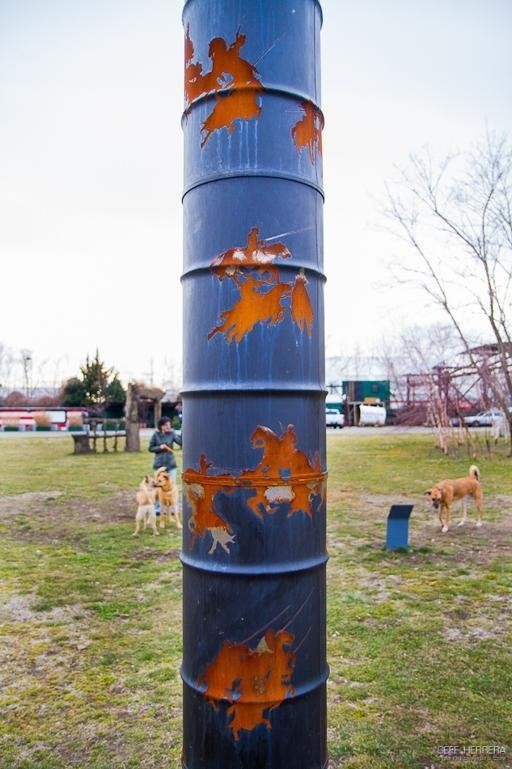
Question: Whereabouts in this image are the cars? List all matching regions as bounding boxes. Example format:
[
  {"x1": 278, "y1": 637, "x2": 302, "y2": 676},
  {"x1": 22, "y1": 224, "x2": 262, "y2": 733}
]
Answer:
[{"x1": 463, "y1": 410, "x2": 502, "y2": 426}]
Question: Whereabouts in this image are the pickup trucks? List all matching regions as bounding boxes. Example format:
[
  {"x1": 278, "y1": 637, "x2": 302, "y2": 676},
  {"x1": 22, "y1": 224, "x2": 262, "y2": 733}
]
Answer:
[{"x1": 325, "y1": 409, "x2": 345, "y2": 428}]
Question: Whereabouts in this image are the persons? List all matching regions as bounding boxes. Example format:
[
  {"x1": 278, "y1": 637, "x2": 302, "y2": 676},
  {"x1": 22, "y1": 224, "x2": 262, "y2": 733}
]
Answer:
[{"x1": 147, "y1": 414, "x2": 184, "y2": 517}]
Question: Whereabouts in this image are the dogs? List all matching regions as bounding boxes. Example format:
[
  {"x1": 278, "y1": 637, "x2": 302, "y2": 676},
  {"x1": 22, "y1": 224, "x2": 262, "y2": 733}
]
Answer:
[
  {"x1": 154, "y1": 465, "x2": 181, "y2": 530},
  {"x1": 131, "y1": 474, "x2": 159, "y2": 538},
  {"x1": 424, "y1": 464, "x2": 485, "y2": 533}
]
